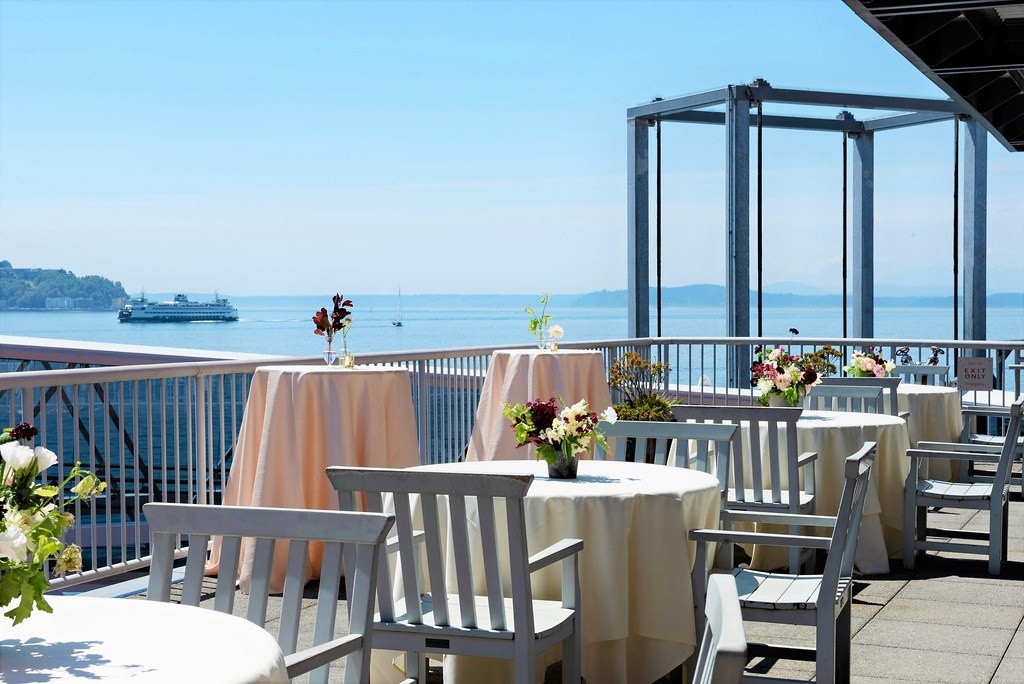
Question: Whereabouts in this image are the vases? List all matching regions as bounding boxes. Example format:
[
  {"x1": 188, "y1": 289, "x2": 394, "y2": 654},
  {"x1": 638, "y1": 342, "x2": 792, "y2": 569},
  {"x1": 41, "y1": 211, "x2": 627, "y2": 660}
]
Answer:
[
  {"x1": 339, "y1": 350, "x2": 354, "y2": 366},
  {"x1": 322, "y1": 336, "x2": 338, "y2": 365},
  {"x1": 853, "y1": 372, "x2": 876, "y2": 378},
  {"x1": 625, "y1": 418, "x2": 678, "y2": 466},
  {"x1": 549, "y1": 342, "x2": 558, "y2": 352},
  {"x1": 768, "y1": 391, "x2": 791, "y2": 407},
  {"x1": 548, "y1": 450, "x2": 579, "y2": 479}
]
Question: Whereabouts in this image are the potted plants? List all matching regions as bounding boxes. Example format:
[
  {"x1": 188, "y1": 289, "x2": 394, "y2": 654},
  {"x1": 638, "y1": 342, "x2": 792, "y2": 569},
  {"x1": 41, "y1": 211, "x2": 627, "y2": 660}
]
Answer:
[{"x1": 522, "y1": 290, "x2": 552, "y2": 350}]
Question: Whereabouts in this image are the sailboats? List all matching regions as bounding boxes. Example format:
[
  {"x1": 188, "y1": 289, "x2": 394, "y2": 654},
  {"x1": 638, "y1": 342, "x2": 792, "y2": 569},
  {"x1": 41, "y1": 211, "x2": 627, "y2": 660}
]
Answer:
[{"x1": 392, "y1": 287, "x2": 403, "y2": 326}]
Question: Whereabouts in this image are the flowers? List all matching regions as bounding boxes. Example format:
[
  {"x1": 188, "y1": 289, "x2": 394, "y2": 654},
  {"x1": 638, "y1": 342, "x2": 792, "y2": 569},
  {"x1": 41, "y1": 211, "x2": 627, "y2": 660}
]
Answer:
[
  {"x1": 0, "y1": 421, "x2": 107, "y2": 627},
  {"x1": 750, "y1": 345, "x2": 824, "y2": 407},
  {"x1": 843, "y1": 345, "x2": 896, "y2": 378},
  {"x1": 546, "y1": 325, "x2": 564, "y2": 344},
  {"x1": 340, "y1": 318, "x2": 352, "y2": 356},
  {"x1": 603, "y1": 353, "x2": 685, "y2": 422},
  {"x1": 501, "y1": 396, "x2": 617, "y2": 463},
  {"x1": 312, "y1": 292, "x2": 354, "y2": 363}
]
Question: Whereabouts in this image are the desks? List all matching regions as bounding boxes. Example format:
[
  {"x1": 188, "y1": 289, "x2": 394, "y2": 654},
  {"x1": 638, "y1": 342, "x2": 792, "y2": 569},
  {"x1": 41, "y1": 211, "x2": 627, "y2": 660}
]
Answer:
[
  {"x1": 204, "y1": 364, "x2": 423, "y2": 595},
  {"x1": 463, "y1": 349, "x2": 611, "y2": 461},
  {"x1": 374, "y1": 458, "x2": 721, "y2": 684},
  {"x1": 802, "y1": 381, "x2": 964, "y2": 483},
  {"x1": 666, "y1": 411, "x2": 916, "y2": 576},
  {"x1": 0, "y1": 595, "x2": 290, "y2": 684}
]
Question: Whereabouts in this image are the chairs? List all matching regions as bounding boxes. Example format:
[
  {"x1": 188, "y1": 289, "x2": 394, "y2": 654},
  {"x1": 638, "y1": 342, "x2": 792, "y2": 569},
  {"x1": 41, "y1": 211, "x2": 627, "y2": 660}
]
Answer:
[{"x1": 143, "y1": 365, "x2": 1024, "y2": 684}]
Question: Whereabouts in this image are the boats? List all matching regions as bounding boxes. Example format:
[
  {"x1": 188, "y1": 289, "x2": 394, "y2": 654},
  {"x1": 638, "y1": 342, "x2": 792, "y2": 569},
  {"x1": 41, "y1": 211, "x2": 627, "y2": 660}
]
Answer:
[{"x1": 118, "y1": 293, "x2": 239, "y2": 323}]
